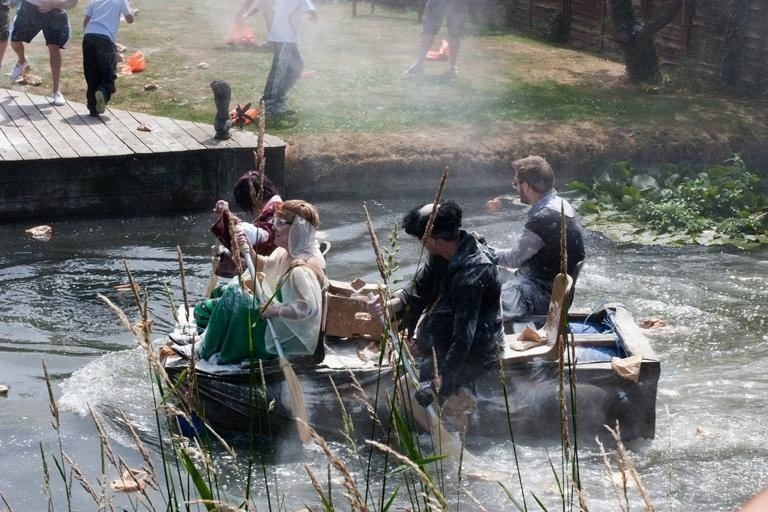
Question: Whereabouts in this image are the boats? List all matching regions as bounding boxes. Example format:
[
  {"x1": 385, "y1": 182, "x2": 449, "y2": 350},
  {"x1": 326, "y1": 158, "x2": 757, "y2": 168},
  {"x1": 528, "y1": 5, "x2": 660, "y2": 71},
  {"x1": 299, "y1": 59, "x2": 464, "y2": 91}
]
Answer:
[{"x1": 163, "y1": 301, "x2": 660, "y2": 447}]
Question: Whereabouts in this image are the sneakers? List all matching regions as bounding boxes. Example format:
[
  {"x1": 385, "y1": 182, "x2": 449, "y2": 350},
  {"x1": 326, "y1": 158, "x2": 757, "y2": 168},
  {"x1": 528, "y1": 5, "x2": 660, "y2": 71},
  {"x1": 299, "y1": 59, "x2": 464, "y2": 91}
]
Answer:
[
  {"x1": 54, "y1": 93, "x2": 66, "y2": 107},
  {"x1": 89, "y1": 91, "x2": 105, "y2": 117},
  {"x1": 9, "y1": 58, "x2": 28, "y2": 78},
  {"x1": 169, "y1": 333, "x2": 203, "y2": 361}
]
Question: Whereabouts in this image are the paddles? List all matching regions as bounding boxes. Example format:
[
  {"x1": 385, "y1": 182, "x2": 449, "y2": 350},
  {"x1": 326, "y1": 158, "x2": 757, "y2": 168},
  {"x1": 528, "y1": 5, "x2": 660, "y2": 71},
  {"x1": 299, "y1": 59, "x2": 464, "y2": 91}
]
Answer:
[
  {"x1": 368, "y1": 291, "x2": 454, "y2": 448},
  {"x1": 240, "y1": 239, "x2": 311, "y2": 444}
]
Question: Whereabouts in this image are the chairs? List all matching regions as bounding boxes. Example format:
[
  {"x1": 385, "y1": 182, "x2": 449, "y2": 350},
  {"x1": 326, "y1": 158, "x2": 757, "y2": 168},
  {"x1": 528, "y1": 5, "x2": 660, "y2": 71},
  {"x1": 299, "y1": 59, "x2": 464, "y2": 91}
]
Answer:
[{"x1": 503, "y1": 273, "x2": 575, "y2": 368}]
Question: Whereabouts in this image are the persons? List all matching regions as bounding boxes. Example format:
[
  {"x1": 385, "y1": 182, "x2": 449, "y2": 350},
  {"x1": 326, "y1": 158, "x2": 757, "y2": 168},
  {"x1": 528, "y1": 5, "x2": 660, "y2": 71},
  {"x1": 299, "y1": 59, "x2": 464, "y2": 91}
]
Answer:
[
  {"x1": 236, "y1": 0, "x2": 276, "y2": 48},
  {"x1": 82, "y1": 1, "x2": 139, "y2": 117},
  {"x1": 404, "y1": 1, "x2": 468, "y2": 76},
  {"x1": 368, "y1": 201, "x2": 502, "y2": 441},
  {"x1": 475, "y1": 155, "x2": 584, "y2": 319},
  {"x1": 170, "y1": 199, "x2": 323, "y2": 364},
  {"x1": 260, "y1": 0, "x2": 319, "y2": 115},
  {"x1": 193, "y1": 171, "x2": 284, "y2": 335},
  {"x1": 8, "y1": 0, "x2": 78, "y2": 105},
  {"x1": 0, "y1": 0, "x2": 9, "y2": 67}
]
